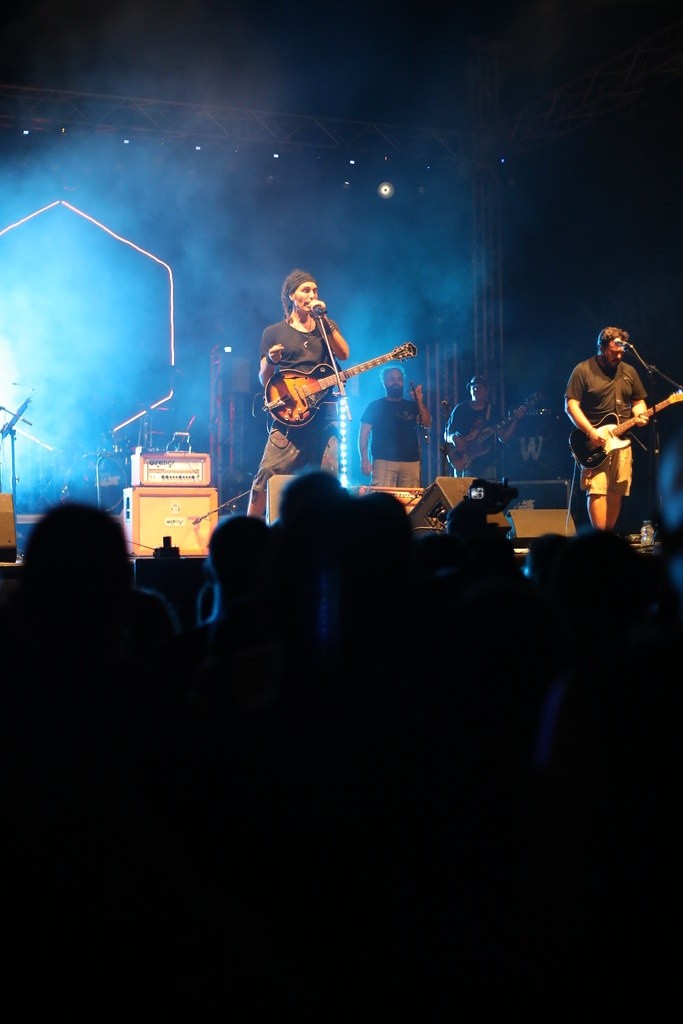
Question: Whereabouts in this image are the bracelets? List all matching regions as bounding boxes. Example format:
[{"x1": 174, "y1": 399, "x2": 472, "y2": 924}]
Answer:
[
  {"x1": 266, "y1": 352, "x2": 279, "y2": 365},
  {"x1": 323, "y1": 319, "x2": 338, "y2": 335}
]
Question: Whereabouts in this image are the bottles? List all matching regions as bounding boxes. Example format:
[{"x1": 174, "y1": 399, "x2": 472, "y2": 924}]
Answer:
[
  {"x1": 131, "y1": 446, "x2": 142, "y2": 487},
  {"x1": 640, "y1": 520, "x2": 655, "y2": 553}
]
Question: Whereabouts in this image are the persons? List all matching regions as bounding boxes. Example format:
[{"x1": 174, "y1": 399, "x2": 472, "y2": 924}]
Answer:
[
  {"x1": 359, "y1": 367, "x2": 432, "y2": 488},
  {"x1": 443, "y1": 375, "x2": 524, "y2": 483},
  {"x1": 247, "y1": 270, "x2": 349, "y2": 517},
  {"x1": 0, "y1": 471, "x2": 683, "y2": 1023},
  {"x1": 564, "y1": 327, "x2": 649, "y2": 530}
]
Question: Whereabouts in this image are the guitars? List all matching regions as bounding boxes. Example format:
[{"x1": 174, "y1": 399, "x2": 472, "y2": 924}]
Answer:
[
  {"x1": 444, "y1": 392, "x2": 544, "y2": 471},
  {"x1": 264, "y1": 341, "x2": 417, "y2": 429},
  {"x1": 568, "y1": 389, "x2": 683, "y2": 470}
]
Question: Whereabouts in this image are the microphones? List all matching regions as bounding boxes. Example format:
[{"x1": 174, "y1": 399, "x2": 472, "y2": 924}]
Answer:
[
  {"x1": 442, "y1": 400, "x2": 447, "y2": 407},
  {"x1": 193, "y1": 518, "x2": 201, "y2": 525},
  {"x1": 313, "y1": 306, "x2": 327, "y2": 314},
  {"x1": 614, "y1": 338, "x2": 633, "y2": 348}
]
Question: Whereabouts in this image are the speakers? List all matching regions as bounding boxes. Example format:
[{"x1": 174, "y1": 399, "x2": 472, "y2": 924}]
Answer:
[
  {"x1": 0, "y1": 493, "x2": 17, "y2": 563},
  {"x1": 406, "y1": 475, "x2": 512, "y2": 538},
  {"x1": 266, "y1": 474, "x2": 295, "y2": 524},
  {"x1": 222, "y1": 358, "x2": 250, "y2": 396},
  {"x1": 501, "y1": 412, "x2": 571, "y2": 480},
  {"x1": 123, "y1": 486, "x2": 218, "y2": 555},
  {"x1": 505, "y1": 509, "x2": 577, "y2": 549}
]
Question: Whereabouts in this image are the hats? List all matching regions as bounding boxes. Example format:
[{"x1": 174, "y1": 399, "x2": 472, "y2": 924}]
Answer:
[{"x1": 465, "y1": 377, "x2": 490, "y2": 390}]
